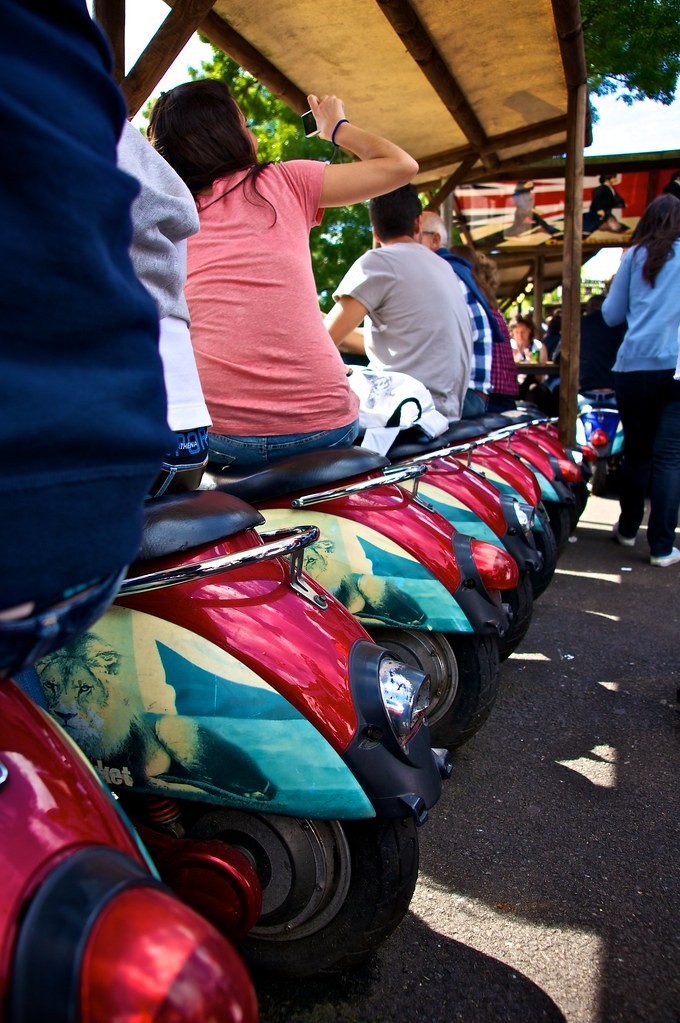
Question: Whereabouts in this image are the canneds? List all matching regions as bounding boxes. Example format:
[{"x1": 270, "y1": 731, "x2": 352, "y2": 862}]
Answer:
[{"x1": 532, "y1": 349, "x2": 539, "y2": 363}]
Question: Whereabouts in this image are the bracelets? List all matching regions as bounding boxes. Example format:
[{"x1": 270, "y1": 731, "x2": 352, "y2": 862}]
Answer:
[{"x1": 332, "y1": 119, "x2": 349, "y2": 147}]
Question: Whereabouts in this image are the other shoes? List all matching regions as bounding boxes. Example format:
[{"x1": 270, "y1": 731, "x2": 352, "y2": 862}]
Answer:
[
  {"x1": 651, "y1": 547, "x2": 680, "y2": 567},
  {"x1": 614, "y1": 522, "x2": 635, "y2": 547}
]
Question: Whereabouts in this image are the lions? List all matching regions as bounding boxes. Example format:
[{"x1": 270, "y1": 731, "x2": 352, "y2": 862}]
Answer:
[
  {"x1": 30, "y1": 629, "x2": 282, "y2": 801},
  {"x1": 277, "y1": 533, "x2": 428, "y2": 629}
]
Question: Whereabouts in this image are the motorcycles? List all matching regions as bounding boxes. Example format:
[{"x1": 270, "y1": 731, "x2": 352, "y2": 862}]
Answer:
[
  {"x1": 1, "y1": 564, "x2": 271, "y2": 1022},
  {"x1": 17, "y1": 485, "x2": 454, "y2": 999},
  {"x1": 217, "y1": 394, "x2": 611, "y2": 761}
]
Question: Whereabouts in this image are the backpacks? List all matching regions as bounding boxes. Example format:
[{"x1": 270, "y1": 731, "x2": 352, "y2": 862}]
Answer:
[{"x1": 344, "y1": 364, "x2": 450, "y2": 458}]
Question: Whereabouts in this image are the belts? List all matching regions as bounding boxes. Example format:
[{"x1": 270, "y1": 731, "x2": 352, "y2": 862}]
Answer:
[
  {"x1": 473, "y1": 390, "x2": 489, "y2": 401},
  {"x1": 0, "y1": 568, "x2": 122, "y2": 653},
  {"x1": 147, "y1": 459, "x2": 210, "y2": 498}
]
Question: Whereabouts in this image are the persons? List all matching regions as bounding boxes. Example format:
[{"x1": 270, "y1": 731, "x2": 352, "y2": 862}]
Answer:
[
  {"x1": 601, "y1": 195, "x2": 680, "y2": 567},
  {"x1": 320, "y1": 183, "x2": 472, "y2": 424},
  {"x1": 421, "y1": 211, "x2": 506, "y2": 420},
  {"x1": 506, "y1": 295, "x2": 617, "y2": 411},
  {"x1": 146, "y1": 78, "x2": 419, "y2": 465},
  {"x1": 448, "y1": 245, "x2": 519, "y2": 414},
  {"x1": 117, "y1": 119, "x2": 213, "y2": 501},
  {"x1": 0, "y1": 0, "x2": 169, "y2": 687}
]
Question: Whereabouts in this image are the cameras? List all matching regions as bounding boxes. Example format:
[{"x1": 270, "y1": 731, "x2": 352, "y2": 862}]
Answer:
[{"x1": 300, "y1": 110, "x2": 320, "y2": 138}]
